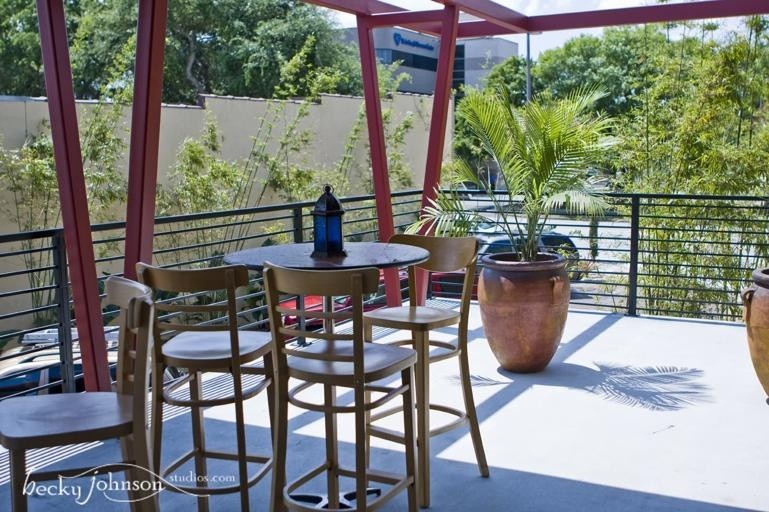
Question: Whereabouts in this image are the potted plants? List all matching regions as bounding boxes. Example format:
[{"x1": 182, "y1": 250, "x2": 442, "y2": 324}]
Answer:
[{"x1": 400, "y1": 78, "x2": 626, "y2": 373}]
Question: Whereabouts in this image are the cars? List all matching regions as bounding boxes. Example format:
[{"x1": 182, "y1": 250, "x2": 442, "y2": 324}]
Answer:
[{"x1": 277, "y1": 177, "x2": 583, "y2": 340}]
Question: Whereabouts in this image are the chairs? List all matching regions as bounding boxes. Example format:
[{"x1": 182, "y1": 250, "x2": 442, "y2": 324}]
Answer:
[{"x1": 1, "y1": 274, "x2": 156, "y2": 511}]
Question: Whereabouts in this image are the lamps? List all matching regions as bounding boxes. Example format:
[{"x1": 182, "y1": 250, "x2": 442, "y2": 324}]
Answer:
[{"x1": 306, "y1": 183, "x2": 349, "y2": 261}]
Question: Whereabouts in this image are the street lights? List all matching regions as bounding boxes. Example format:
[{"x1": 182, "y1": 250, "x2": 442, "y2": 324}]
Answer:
[{"x1": 520, "y1": 29, "x2": 546, "y2": 197}]
